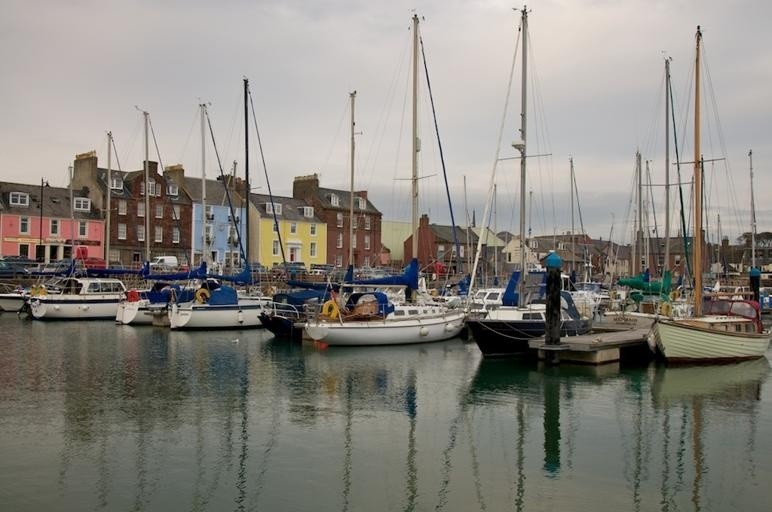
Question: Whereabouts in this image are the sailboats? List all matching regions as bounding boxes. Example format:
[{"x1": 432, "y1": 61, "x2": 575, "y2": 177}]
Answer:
[
  {"x1": 26, "y1": 163, "x2": 159, "y2": 323},
  {"x1": 256, "y1": 13, "x2": 470, "y2": 348},
  {"x1": 463, "y1": 4, "x2": 597, "y2": 363},
  {"x1": 566, "y1": 53, "x2": 772, "y2": 345},
  {"x1": 147, "y1": 102, "x2": 289, "y2": 331},
  {"x1": 644, "y1": 23, "x2": 771, "y2": 369}
]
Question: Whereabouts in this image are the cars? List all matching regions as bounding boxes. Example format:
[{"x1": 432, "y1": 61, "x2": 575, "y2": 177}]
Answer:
[{"x1": 0, "y1": 255, "x2": 87, "y2": 279}]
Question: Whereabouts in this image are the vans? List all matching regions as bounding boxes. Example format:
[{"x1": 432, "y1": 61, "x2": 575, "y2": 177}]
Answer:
[{"x1": 142, "y1": 255, "x2": 179, "y2": 272}]
